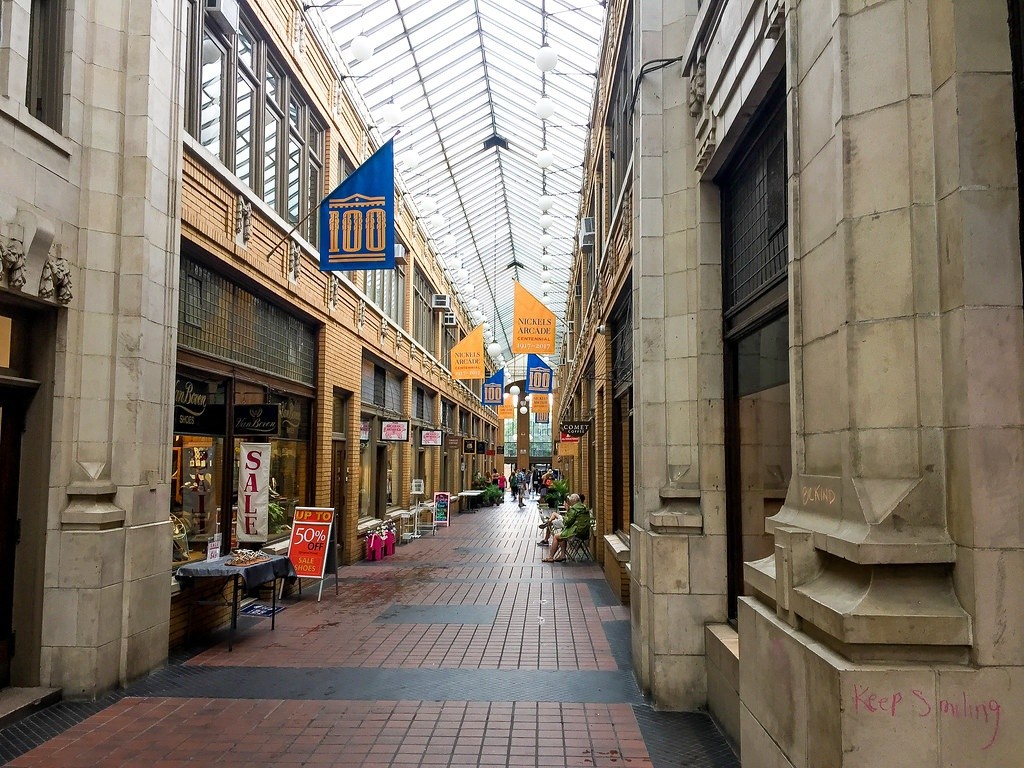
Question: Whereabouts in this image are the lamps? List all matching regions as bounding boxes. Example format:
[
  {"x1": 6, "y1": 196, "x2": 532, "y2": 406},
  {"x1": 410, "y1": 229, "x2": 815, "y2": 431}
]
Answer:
[
  {"x1": 346, "y1": 24, "x2": 531, "y2": 428},
  {"x1": 533, "y1": 33, "x2": 562, "y2": 364}
]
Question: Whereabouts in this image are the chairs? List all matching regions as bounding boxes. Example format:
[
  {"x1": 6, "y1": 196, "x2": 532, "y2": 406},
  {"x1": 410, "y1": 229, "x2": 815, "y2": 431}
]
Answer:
[{"x1": 543, "y1": 507, "x2": 593, "y2": 562}]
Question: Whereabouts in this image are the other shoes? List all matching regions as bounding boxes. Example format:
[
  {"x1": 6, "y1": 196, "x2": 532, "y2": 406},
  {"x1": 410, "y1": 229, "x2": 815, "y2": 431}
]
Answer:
[
  {"x1": 542, "y1": 556, "x2": 554, "y2": 562},
  {"x1": 539, "y1": 523, "x2": 547, "y2": 529},
  {"x1": 555, "y1": 555, "x2": 567, "y2": 562},
  {"x1": 537, "y1": 540, "x2": 549, "y2": 546}
]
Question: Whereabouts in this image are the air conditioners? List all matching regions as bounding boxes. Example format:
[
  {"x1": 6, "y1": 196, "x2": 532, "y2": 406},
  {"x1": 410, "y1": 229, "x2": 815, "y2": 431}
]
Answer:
[
  {"x1": 431, "y1": 289, "x2": 457, "y2": 332},
  {"x1": 394, "y1": 240, "x2": 409, "y2": 267},
  {"x1": 576, "y1": 216, "x2": 596, "y2": 256}
]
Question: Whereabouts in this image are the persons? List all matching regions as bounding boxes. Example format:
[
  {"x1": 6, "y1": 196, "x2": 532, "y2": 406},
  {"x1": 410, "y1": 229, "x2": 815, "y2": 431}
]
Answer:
[{"x1": 474, "y1": 465, "x2": 590, "y2": 563}]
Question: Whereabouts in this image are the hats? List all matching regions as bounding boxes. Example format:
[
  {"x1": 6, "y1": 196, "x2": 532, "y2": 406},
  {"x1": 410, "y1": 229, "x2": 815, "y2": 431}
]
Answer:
[{"x1": 548, "y1": 469, "x2": 553, "y2": 474}]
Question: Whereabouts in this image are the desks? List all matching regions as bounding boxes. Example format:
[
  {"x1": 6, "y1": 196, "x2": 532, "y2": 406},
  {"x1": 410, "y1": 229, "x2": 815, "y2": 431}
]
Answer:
[
  {"x1": 457, "y1": 486, "x2": 487, "y2": 519},
  {"x1": 176, "y1": 550, "x2": 287, "y2": 654}
]
